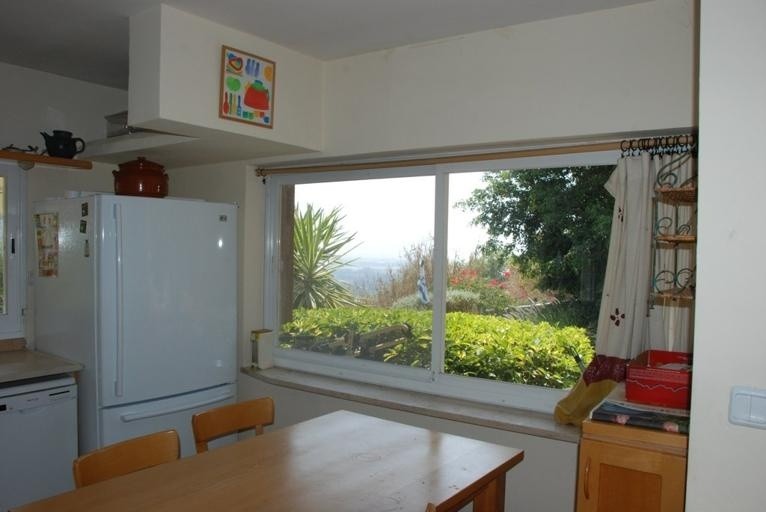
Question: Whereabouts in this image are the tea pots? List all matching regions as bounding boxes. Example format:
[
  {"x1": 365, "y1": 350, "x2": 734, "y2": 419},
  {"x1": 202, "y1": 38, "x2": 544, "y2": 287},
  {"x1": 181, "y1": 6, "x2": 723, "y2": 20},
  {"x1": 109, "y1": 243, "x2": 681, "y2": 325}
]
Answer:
[{"x1": 39, "y1": 129, "x2": 85, "y2": 158}]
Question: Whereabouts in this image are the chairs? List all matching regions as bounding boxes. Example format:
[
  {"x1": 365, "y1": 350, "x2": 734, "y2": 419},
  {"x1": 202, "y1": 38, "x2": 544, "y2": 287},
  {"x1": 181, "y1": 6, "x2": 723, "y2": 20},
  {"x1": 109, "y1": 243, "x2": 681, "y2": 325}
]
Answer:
[{"x1": 72, "y1": 395, "x2": 276, "y2": 489}]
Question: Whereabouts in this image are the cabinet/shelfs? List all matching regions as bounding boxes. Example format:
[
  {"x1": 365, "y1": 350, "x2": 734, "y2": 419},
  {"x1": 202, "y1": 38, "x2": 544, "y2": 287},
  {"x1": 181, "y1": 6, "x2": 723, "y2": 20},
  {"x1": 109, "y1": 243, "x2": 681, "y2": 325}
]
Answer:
[
  {"x1": 574, "y1": 416, "x2": 688, "y2": 511},
  {"x1": 647, "y1": 142, "x2": 695, "y2": 311}
]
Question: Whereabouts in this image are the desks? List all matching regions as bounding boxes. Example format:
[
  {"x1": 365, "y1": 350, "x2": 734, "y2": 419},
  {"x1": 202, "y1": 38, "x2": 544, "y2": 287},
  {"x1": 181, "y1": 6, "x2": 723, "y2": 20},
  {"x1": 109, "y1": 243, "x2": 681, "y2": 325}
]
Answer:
[{"x1": 0, "y1": 407, "x2": 526, "y2": 510}]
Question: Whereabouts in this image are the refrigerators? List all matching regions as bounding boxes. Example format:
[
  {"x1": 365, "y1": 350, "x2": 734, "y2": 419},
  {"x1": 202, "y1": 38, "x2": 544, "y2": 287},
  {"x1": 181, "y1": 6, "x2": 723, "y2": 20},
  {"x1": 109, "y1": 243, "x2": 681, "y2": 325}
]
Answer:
[
  {"x1": 27, "y1": 189, "x2": 238, "y2": 457},
  {"x1": 0, "y1": 372, "x2": 79, "y2": 511}
]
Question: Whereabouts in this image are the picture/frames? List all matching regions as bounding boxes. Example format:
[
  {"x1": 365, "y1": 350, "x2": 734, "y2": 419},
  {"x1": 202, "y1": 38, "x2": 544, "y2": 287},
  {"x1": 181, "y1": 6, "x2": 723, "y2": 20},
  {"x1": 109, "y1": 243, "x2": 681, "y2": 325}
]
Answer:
[{"x1": 217, "y1": 44, "x2": 277, "y2": 131}]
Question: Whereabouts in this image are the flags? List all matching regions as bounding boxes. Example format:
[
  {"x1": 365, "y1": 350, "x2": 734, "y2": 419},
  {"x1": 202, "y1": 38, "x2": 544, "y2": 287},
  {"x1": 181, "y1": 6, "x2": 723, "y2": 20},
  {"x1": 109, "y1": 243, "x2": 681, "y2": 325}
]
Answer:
[{"x1": 417, "y1": 257, "x2": 430, "y2": 304}]
